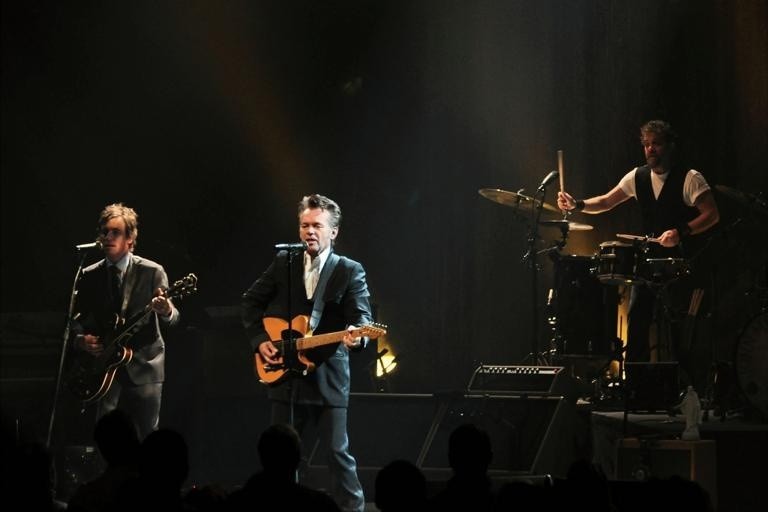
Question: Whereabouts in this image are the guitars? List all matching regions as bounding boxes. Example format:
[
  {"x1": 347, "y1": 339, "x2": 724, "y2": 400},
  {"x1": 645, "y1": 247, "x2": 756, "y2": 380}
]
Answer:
[
  {"x1": 255, "y1": 314, "x2": 388, "y2": 385},
  {"x1": 60, "y1": 272, "x2": 198, "y2": 403}
]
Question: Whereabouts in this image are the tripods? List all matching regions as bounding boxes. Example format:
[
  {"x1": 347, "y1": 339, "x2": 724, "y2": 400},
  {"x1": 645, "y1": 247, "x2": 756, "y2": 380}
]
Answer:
[{"x1": 576, "y1": 264, "x2": 676, "y2": 417}]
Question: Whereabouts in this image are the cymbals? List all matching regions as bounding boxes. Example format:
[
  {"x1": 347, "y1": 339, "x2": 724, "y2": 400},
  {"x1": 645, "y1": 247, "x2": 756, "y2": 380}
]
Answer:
[
  {"x1": 541, "y1": 220, "x2": 594, "y2": 231},
  {"x1": 479, "y1": 189, "x2": 560, "y2": 217}
]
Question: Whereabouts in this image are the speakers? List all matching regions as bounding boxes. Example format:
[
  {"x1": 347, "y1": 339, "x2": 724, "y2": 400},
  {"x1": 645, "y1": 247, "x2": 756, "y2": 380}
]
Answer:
[
  {"x1": 306, "y1": 393, "x2": 444, "y2": 486},
  {"x1": 419, "y1": 395, "x2": 567, "y2": 486}
]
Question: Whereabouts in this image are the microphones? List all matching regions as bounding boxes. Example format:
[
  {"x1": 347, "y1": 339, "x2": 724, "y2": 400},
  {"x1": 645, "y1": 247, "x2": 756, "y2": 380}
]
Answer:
[
  {"x1": 76, "y1": 240, "x2": 103, "y2": 251},
  {"x1": 275, "y1": 240, "x2": 309, "y2": 252},
  {"x1": 537, "y1": 171, "x2": 558, "y2": 191}
]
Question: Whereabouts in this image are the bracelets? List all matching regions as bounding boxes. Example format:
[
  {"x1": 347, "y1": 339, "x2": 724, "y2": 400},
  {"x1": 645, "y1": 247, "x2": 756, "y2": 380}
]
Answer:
[
  {"x1": 675, "y1": 223, "x2": 692, "y2": 241},
  {"x1": 574, "y1": 199, "x2": 585, "y2": 212}
]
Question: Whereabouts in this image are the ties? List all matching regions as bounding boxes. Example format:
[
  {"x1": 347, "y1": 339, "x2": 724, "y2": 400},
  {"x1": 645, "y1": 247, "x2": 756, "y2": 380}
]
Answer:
[{"x1": 112, "y1": 270, "x2": 121, "y2": 312}]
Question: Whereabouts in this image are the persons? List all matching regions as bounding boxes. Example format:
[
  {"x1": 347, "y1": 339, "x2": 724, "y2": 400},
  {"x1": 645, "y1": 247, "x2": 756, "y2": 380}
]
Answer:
[
  {"x1": 63, "y1": 201, "x2": 180, "y2": 442},
  {"x1": 239, "y1": 192, "x2": 376, "y2": 511},
  {"x1": 555, "y1": 118, "x2": 721, "y2": 410}
]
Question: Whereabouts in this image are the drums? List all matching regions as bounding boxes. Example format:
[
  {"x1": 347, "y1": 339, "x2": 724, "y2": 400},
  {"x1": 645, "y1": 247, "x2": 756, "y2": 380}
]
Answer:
[
  {"x1": 733, "y1": 310, "x2": 767, "y2": 412},
  {"x1": 552, "y1": 254, "x2": 654, "y2": 362},
  {"x1": 596, "y1": 240, "x2": 645, "y2": 287}
]
